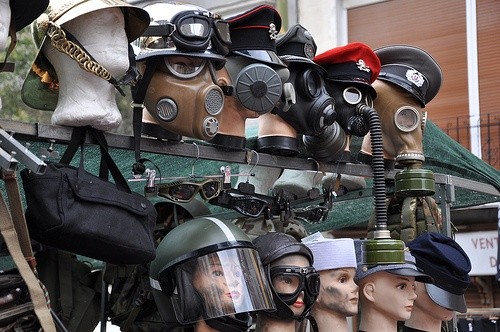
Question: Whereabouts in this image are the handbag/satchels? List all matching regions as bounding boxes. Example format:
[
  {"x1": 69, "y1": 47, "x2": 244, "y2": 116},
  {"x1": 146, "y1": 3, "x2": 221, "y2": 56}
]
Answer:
[
  {"x1": 0, "y1": 268, "x2": 50, "y2": 332},
  {"x1": 20, "y1": 125, "x2": 158, "y2": 266}
]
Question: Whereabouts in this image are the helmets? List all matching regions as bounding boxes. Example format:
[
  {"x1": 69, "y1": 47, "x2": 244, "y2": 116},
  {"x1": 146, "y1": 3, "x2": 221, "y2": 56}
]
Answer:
[
  {"x1": 154, "y1": 197, "x2": 211, "y2": 224},
  {"x1": 149, "y1": 216, "x2": 277, "y2": 324},
  {"x1": 251, "y1": 232, "x2": 314, "y2": 267},
  {"x1": 130, "y1": 2, "x2": 227, "y2": 71}
]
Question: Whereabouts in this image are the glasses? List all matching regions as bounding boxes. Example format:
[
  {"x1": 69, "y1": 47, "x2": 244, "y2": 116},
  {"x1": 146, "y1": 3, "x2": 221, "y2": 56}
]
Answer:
[
  {"x1": 270, "y1": 265, "x2": 320, "y2": 305},
  {"x1": 141, "y1": 10, "x2": 232, "y2": 56},
  {"x1": 209, "y1": 188, "x2": 276, "y2": 218},
  {"x1": 158, "y1": 178, "x2": 221, "y2": 203}
]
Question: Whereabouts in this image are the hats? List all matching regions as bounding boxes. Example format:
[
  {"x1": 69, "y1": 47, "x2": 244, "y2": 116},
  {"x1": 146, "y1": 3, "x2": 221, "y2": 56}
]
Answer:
[
  {"x1": 406, "y1": 232, "x2": 472, "y2": 313},
  {"x1": 223, "y1": 4, "x2": 288, "y2": 68},
  {"x1": 372, "y1": 44, "x2": 442, "y2": 108},
  {"x1": 274, "y1": 24, "x2": 329, "y2": 79},
  {"x1": 354, "y1": 239, "x2": 434, "y2": 284},
  {"x1": 300, "y1": 230, "x2": 357, "y2": 272},
  {"x1": 21, "y1": 0, "x2": 150, "y2": 112},
  {"x1": 314, "y1": 42, "x2": 381, "y2": 100},
  {"x1": 9, "y1": 0, "x2": 49, "y2": 37}
]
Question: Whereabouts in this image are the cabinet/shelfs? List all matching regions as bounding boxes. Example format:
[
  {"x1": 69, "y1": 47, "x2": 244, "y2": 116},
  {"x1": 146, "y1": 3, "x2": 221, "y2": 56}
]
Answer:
[{"x1": 0, "y1": 110, "x2": 500, "y2": 332}]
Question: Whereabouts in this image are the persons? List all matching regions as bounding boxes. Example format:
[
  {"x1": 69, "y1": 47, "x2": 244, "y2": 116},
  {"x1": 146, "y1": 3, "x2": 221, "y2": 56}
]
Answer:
[
  {"x1": 361, "y1": 43, "x2": 442, "y2": 160},
  {"x1": 130, "y1": 2, "x2": 227, "y2": 142},
  {"x1": 357, "y1": 239, "x2": 431, "y2": 332},
  {"x1": 303, "y1": 237, "x2": 359, "y2": 332},
  {"x1": 302, "y1": 43, "x2": 381, "y2": 162},
  {"x1": 0, "y1": 0, "x2": 49, "y2": 72},
  {"x1": 251, "y1": 232, "x2": 321, "y2": 332},
  {"x1": 18, "y1": 1, "x2": 151, "y2": 134},
  {"x1": 147, "y1": 215, "x2": 276, "y2": 332},
  {"x1": 405, "y1": 230, "x2": 472, "y2": 332},
  {"x1": 255, "y1": 22, "x2": 317, "y2": 157},
  {"x1": 209, "y1": 3, "x2": 282, "y2": 152}
]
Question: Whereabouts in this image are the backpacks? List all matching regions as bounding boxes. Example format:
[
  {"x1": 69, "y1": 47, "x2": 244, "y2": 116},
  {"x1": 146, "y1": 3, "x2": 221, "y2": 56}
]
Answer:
[
  {"x1": 34, "y1": 248, "x2": 102, "y2": 332},
  {"x1": 231, "y1": 214, "x2": 308, "y2": 241},
  {"x1": 368, "y1": 194, "x2": 456, "y2": 238},
  {"x1": 102, "y1": 262, "x2": 177, "y2": 332}
]
